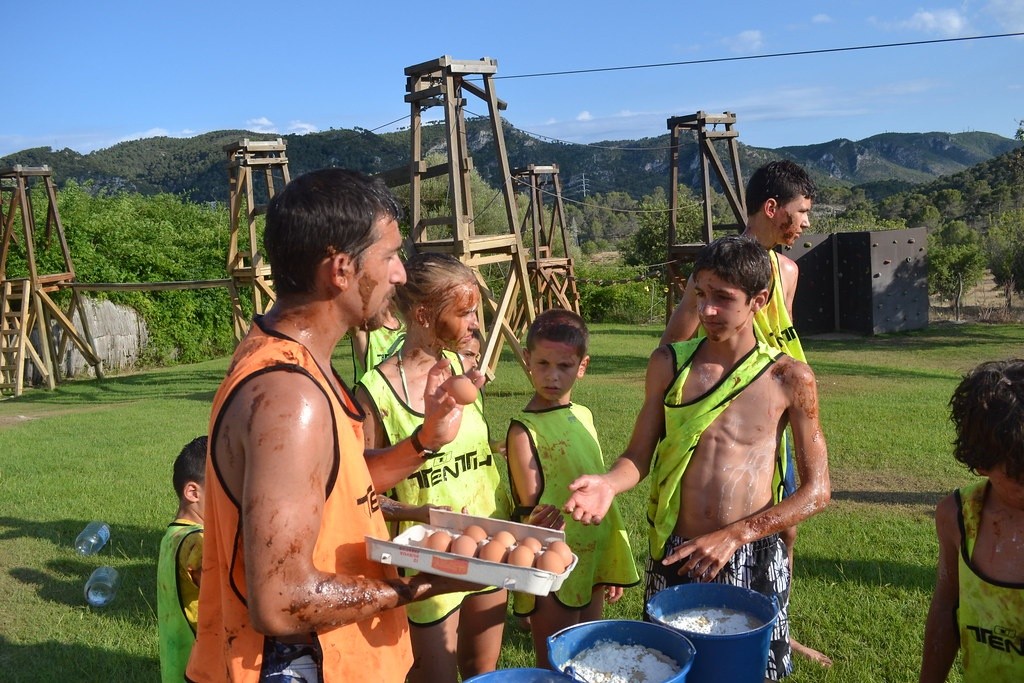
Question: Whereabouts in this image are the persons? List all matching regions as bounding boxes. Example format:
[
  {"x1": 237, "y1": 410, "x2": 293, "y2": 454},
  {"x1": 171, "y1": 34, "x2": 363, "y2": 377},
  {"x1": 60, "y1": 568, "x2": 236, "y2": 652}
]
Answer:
[
  {"x1": 157, "y1": 435, "x2": 208, "y2": 683},
  {"x1": 351, "y1": 252, "x2": 642, "y2": 683},
  {"x1": 918, "y1": 359, "x2": 1024, "y2": 683},
  {"x1": 566, "y1": 236, "x2": 831, "y2": 683},
  {"x1": 186, "y1": 167, "x2": 492, "y2": 683},
  {"x1": 655, "y1": 160, "x2": 834, "y2": 670}
]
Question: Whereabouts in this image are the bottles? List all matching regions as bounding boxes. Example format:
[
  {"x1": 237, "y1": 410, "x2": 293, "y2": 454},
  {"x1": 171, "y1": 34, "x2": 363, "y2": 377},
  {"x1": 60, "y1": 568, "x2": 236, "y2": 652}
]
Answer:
[
  {"x1": 75, "y1": 522, "x2": 110, "y2": 557},
  {"x1": 84, "y1": 566, "x2": 119, "y2": 607}
]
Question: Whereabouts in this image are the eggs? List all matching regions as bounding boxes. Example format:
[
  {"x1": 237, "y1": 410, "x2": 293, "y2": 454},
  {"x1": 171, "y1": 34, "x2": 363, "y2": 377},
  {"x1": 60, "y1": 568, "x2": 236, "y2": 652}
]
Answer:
[
  {"x1": 447, "y1": 379, "x2": 477, "y2": 405},
  {"x1": 425, "y1": 525, "x2": 574, "y2": 575}
]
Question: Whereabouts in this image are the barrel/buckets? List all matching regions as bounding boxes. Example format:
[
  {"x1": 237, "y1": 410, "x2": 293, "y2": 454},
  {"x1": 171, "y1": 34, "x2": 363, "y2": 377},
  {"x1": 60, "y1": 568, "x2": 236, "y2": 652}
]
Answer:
[{"x1": 462, "y1": 582, "x2": 780, "y2": 683}]
